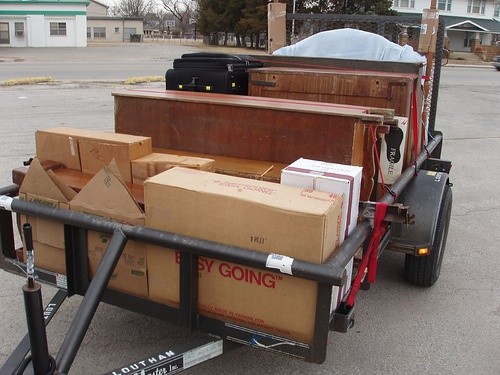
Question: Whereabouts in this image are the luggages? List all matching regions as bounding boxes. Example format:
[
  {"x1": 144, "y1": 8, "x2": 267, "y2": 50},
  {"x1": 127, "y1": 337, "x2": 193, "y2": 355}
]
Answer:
[{"x1": 164, "y1": 51, "x2": 266, "y2": 97}]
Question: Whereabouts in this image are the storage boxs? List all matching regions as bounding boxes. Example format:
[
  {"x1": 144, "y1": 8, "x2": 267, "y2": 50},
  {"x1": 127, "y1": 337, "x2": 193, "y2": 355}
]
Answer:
[
  {"x1": 143, "y1": 165, "x2": 345, "y2": 346},
  {"x1": 34, "y1": 125, "x2": 153, "y2": 182},
  {"x1": 281, "y1": 156, "x2": 364, "y2": 311},
  {"x1": 130, "y1": 151, "x2": 215, "y2": 187}
]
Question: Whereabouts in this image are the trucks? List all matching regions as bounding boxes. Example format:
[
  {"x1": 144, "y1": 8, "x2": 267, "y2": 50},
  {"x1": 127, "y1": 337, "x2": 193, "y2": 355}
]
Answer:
[{"x1": 1, "y1": 0, "x2": 454, "y2": 375}]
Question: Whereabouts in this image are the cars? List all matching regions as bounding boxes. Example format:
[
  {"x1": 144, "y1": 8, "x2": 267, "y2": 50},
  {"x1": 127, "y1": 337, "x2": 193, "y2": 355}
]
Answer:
[{"x1": 491, "y1": 54, "x2": 500, "y2": 72}]
